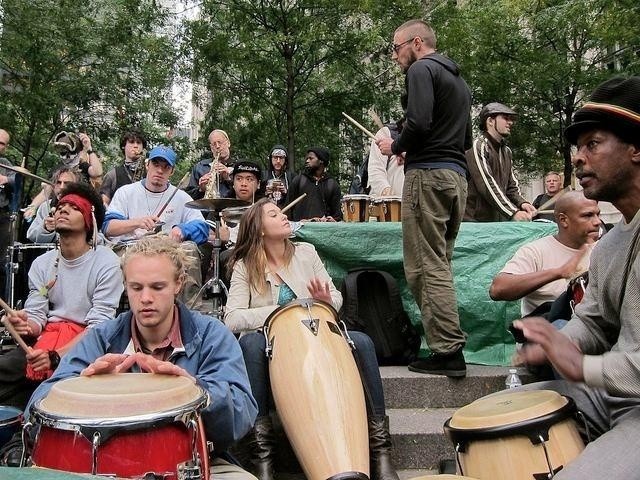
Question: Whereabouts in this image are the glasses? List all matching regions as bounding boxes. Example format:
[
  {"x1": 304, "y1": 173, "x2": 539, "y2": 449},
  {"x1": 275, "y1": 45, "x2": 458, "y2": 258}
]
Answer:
[{"x1": 393, "y1": 36, "x2": 424, "y2": 54}]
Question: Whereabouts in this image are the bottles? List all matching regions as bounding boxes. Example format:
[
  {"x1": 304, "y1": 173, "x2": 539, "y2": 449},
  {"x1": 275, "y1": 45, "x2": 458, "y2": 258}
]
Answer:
[
  {"x1": 368, "y1": 205, "x2": 378, "y2": 222},
  {"x1": 504, "y1": 368, "x2": 522, "y2": 390}
]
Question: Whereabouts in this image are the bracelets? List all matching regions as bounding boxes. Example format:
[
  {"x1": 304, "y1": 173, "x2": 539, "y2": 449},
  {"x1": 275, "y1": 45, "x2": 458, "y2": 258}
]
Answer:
[{"x1": 48, "y1": 350, "x2": 62, "y2": 370}]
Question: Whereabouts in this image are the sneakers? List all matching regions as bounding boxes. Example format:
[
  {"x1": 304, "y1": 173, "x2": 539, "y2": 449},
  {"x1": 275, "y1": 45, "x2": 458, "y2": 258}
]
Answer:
[{"x1": 408, "y1": 347, "x2": 467, "y2": 376}]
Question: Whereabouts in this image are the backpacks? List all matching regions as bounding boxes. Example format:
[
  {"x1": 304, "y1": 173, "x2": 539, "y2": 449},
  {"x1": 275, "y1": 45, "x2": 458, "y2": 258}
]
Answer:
[{"x1": 339, "y1": 267, "x2": 421, "y2": 366}]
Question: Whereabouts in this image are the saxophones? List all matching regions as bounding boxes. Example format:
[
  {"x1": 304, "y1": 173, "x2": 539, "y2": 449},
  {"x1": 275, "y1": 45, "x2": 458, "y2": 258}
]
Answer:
[
  {"x1": 131, "y1": 149, "x2": 146, "y2": 183},
  {"x1": 203, "y1": 152, "x2": 221, "y2": 199}
]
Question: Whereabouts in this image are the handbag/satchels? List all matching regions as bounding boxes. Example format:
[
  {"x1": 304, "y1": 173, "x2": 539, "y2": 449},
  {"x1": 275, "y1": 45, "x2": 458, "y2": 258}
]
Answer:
[{"x1": 349, "y1": 155, "x2": 370, "y2": 194}]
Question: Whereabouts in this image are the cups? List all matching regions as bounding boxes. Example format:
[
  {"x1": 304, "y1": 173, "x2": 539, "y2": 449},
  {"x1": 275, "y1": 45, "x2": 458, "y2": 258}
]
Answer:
[{"x1": 273, "y1": 180, "x2": 282, "y2": 201}]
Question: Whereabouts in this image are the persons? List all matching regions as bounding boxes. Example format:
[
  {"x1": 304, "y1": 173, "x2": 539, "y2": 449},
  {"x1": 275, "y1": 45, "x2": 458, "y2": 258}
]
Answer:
[
  {"x1": 531, "y1": 171, "x2": 562, "y2": 221},
  {"x1": 461, "y1": 101, "x2": 537, "y2": 221},
  {"x1": 375, "y1": 19, "x2": 473, "y2": 377},
  {"x1": 23, "y1": 129, "x2": 343, "y2": 310},
  {"x1": 488, "y1": 189, "x2": 602, "y2": 385},
  {"x1": 1, "y1": 182, "x2": 126, "y2": 413},
  {"x1": 25, "y1": 236, "x2": 258, "y2": 480},
  {"x1": 509, "y1": 75, "x2": 640, "y2": 480},
  {"x1": 223, "y1": 198, "x2": 400, "y2": 479},
  {"x1": 366, "y1": 127, "x2": 406, "y2": 223}
]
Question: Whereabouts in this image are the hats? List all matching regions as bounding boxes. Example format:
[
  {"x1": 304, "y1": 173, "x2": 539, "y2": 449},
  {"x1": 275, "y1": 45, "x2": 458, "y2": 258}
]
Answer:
[
  {"x1": 480, "y1": 102, "x2": 518, "y2": 119},
  {"x1": 233, "y1": 162, "x2": 260, "y2": 178},
  {"x1": 307, "y1": 148, "x2": 329, "y2": 166},
  {"x1": 563, "y1": 76, "x2": 640, "y2": 144},
  {"x1": 269, "y1": 146, "x2": 287, "y2": 157},
  {"x1": 147, "y1": 146, "x2": 177, "y2": 167},
  {"x1": 54, "y1": 131, "x2": 81, "y2": 152}
]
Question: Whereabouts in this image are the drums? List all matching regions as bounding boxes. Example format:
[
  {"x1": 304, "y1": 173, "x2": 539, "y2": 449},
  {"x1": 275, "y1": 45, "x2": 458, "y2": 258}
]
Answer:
[
  {"x1": 13, "y1": 243, "x2": 61, "y2": 310},
  {"x1": 567, "y1": 271, "x2": 588, "y2": 315},
  {"x1": 342, "y1": 195, "x2": 374, "y2": 222},
  {"x1": 444, "y1": 390, "x2": 587, "y2": 480},
  {"x1": 30, "y1": 372, "x2": 209, "y2": 480},
  {"x1": 259, "y1": 297, "x2": 370, "y2": 479},
  {"x1": 1, "y1": 405, "x2": 23, "y2": 466},
  {"x1": 369, "y1": 196, "x2": 401, "y2": 222}
]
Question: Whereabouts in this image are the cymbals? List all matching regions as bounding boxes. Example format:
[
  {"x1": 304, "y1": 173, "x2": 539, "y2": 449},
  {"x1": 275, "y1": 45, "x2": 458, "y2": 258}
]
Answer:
[
  {"x1": 0, "y1": 163, "x2": 55, "y2": 187},
  {"x1": 185, "y1": 198, "x2": 252, "y2": 212}
]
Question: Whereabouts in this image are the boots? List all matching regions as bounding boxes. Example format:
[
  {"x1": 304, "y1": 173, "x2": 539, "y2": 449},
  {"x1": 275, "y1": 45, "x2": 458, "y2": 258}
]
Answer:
[
  {"x1": 247, "y1": 417, "x2": 279, "y2": 480},
  {"x1": 368, "y1": 414, "x2": 400, "y2": 480}
]
